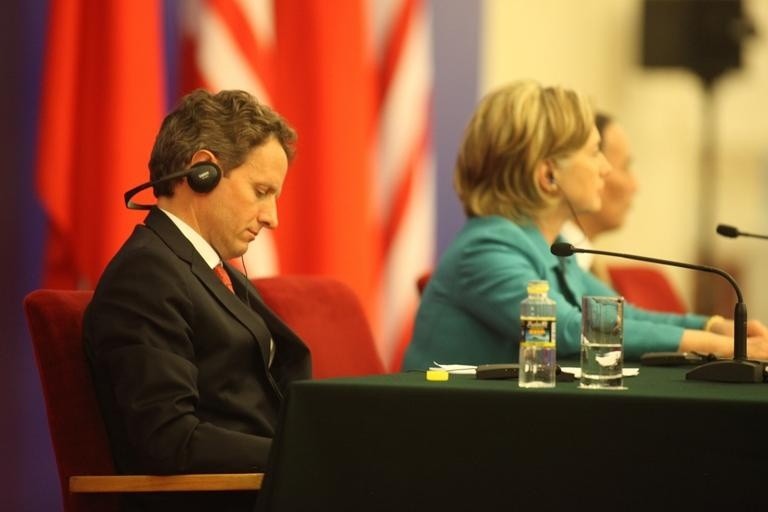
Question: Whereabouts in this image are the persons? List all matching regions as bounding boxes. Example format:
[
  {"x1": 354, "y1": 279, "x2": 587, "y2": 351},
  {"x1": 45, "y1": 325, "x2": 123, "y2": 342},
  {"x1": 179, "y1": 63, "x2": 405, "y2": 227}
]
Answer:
[
  {"x1": 83, "y1": 88, "x2": 312, "y2": 512},
  {"x1": 397, "y1": 76, "x2": 768, "y2": 375},
  {"x1": 557, "y1": 112, "x2": 639, "y2": 288}
]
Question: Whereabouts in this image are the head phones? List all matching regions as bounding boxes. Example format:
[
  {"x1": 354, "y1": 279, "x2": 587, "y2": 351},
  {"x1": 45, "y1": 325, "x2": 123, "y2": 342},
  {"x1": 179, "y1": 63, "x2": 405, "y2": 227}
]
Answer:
[{"x1": 126, "y1": 161, "x2": 221, "y2": 210}]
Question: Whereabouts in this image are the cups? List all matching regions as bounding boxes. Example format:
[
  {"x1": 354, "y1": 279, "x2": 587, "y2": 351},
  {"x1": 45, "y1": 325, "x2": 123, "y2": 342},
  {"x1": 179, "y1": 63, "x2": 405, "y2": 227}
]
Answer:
[{"x1": 578, "y1": 296, "x2": 623, "y2": 389}]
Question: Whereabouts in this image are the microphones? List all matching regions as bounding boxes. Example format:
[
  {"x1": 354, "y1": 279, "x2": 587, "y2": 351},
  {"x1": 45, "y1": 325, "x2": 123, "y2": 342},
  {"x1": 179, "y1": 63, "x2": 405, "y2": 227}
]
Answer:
[
  {"x1": 549, "y1": 242, "x2": 767, "y2": 383},
  {"x1": 716, "y1": 225, "x2": 768, "y2": 240}
]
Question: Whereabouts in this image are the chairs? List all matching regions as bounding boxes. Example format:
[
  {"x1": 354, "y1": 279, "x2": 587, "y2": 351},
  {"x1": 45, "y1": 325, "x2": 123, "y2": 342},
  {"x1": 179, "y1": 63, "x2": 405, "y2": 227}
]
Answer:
[
  {"x1": 20, "y1": 288, "x2": 266, "y2": 510},
  {"x1": 416, "y1": 272, "x2": 432, "y2": 297},
  {"x1": 606, "y1": 263, "x2": 687, "y2": 314},
  {"x1": 250, "y1": 274, "x2": 387, "y2": 379}
]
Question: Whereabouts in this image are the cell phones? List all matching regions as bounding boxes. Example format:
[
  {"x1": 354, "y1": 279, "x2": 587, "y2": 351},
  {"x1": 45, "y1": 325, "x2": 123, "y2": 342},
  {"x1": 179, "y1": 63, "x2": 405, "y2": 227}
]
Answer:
[{"x1": 476, "y1": 363, "x2": 560, "y2": 379}]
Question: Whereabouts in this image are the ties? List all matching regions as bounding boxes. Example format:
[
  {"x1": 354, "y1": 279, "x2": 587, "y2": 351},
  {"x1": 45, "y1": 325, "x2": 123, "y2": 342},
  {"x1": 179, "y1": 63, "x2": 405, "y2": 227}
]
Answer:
[
  {"x1": 213, "y1": 261, "x2": 236, "y2": 298},
  {"x1": 552, "y1": 266, "x2": 583, "y2": 313}
]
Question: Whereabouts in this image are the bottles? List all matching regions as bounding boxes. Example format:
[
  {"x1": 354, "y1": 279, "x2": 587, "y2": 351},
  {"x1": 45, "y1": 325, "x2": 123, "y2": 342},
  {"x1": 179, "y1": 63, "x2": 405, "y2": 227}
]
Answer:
[{"x1": 517, "y1": 280, "x2": 556, "y2": 387}]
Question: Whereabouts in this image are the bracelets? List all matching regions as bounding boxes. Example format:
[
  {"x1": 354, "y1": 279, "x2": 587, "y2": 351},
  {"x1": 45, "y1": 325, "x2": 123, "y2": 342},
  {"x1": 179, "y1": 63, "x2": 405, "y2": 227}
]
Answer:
[{"x1": 703, "y1": 313, "x2": 723, "y2": 332}]
